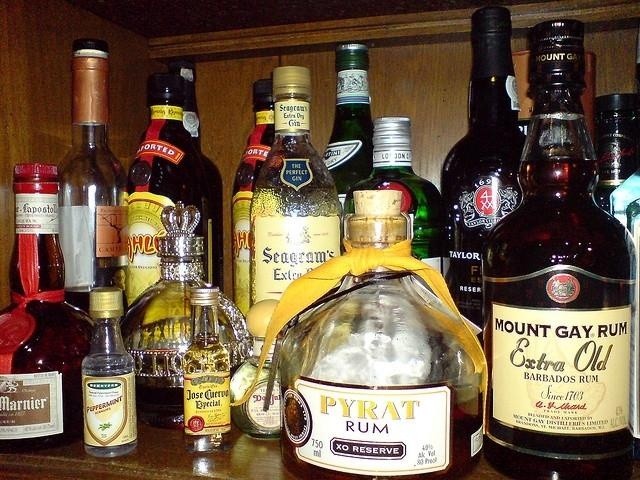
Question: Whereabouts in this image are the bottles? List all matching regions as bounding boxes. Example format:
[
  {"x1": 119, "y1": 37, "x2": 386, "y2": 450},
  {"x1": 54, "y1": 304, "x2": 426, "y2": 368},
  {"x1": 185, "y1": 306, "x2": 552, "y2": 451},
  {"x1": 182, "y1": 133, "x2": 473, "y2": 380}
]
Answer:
[
  {"x1": 121, "y1": 202, "x2": 253, "y2": 426},
  {"x1": 2, "y1": 162, "x2": 91, "y2": 453},
  {"x1": 228, "y1": 297, "x2": 284, "y2": 440},
  {"x1": 233, "y1": 66, "x2": 341, "y2": 315},
  {"x1": 323, "y1": 42, "x2": 375, "y2": 206},
  {"x1": 340, "y1": 116, "x2": 440, "y2": 271},
  {"x1": 182, "y1": 286, "x2": 232, "y2": 454},
  {"x1": 580, "y1": 49, "x2": 597, "y2": 145},
  {"x1": 275, "y1": 188, "x2": 484, "y2": 479},
  {"x1": 485, "y1": 15, "x2": 633, "y2": 480},
  {"x1": 58, "y1": 39, "x2": 130, "y2": 317},
  {"x1": 581, "y1": 89, "x2": 640, "y2": 231},
  {"x1": 81, "y1": 287, "x2": 140, "y2": 462},
  {"x1": 123, "y1": 61, "x2": 222, "y2": 313},
  {"x1": 441, "y1": 6, "x2": 526, "y2": 343}
]
